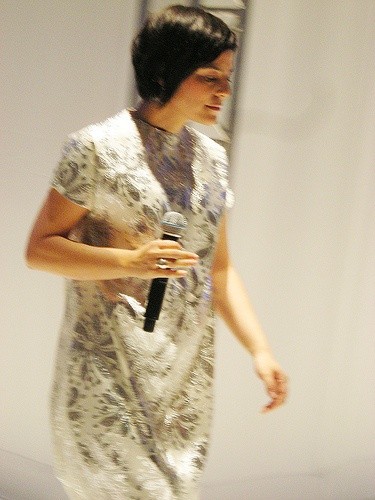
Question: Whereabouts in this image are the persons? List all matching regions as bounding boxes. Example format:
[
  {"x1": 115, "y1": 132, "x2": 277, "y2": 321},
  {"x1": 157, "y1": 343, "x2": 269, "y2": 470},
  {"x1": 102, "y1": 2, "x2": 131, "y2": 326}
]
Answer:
[{"x1": 25, "y1": 5, "x2": 288, "y2": 500}]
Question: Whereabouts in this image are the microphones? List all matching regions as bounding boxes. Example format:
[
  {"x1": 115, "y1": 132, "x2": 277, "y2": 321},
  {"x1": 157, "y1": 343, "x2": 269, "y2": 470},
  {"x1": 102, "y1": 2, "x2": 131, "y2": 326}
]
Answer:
[{"x1": 142, "y1": 211, "x2": 187, "y2": 332}]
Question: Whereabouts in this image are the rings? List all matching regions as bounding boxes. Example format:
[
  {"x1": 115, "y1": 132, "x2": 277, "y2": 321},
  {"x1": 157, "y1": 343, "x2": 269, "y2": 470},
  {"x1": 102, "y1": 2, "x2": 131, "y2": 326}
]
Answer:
[{"x1": 158, "y1": 259, "x2": 169, "y2": 269}]
